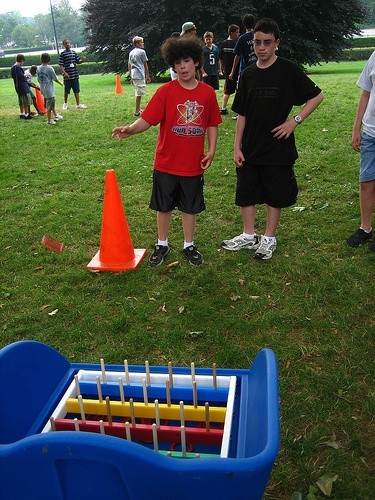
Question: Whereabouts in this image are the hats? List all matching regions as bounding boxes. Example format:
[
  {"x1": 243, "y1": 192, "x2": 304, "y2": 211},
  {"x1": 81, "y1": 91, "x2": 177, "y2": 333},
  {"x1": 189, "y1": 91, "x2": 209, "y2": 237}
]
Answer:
[{"x1": 179, "y1": 21, "x2": 195, "y2": 37}]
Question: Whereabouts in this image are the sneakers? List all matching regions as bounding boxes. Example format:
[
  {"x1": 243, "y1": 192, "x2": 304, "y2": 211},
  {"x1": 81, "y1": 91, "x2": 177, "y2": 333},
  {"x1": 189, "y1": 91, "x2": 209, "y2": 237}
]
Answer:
[
  {"x1": 132, "y1": 111, "x2": 142, "y2": 117},
  {"x1": 47, "y1": 119, "x2": 56, "y2": 125},
  {"x1": 54, "y1": 114, "x2": 62, "y2": 121},
  {"x1": 76, "y1": 105, "x2": 86, "y2": 109},
  {"x1": 148, "y1": 243, "x2": 170, "y2": 268},
  {"x1": 220, "y1": 108, "x2": 229, "y2": 115},
  {"x1": 63, "y1": 103, "x2": 68, "y2": 109},
  {"x1": 255, "y1": 235, "x2": 277, "y2": 260},
  {"x1": 347, "y1": 227, "x2": 373, "y2": 247},
  {"x1": 221, "y1": 233, "x2": 259, "y2": 250},
  {"x1": 181, "y1": 244, "x2": 205, "y2": 267}
]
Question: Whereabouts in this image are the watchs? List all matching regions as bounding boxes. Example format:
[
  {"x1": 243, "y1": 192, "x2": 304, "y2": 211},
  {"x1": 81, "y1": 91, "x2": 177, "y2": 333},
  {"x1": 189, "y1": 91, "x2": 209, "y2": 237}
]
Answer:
[{"x1": 293, "y1": 114, "x2": 303, "y2": 124}]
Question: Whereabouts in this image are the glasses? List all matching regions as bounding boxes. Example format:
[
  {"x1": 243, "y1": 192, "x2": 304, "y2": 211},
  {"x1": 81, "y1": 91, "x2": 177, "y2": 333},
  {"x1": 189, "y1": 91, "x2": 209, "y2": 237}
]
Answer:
[{"x1": 251, "y1": 39, "x2": 275, "y2": 48}]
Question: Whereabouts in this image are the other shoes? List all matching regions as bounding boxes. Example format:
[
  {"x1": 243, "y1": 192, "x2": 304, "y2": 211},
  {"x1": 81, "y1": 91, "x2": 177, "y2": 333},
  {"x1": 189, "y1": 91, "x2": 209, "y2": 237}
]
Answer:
[{"x1": 20, "y1": 115, "x2": 34, "y2": 120}]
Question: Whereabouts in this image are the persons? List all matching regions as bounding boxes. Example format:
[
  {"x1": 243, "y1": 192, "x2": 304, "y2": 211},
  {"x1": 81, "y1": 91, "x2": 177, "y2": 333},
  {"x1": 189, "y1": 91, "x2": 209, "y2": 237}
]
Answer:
[
  {"x1": 10, "y1": 54, "x2": 45, "y2": 120},
  {"x1": 111, "y1": 34, "x2": 222, "y2": 268},
  {"x1": 127, "y1": 36, "x2": 151, "y2": 118},
  {"x1": 221, "y1": 17, "x2": 324, "y2": 260},
  {"x1": 57, "y1": 39, "x2": 87, "y2": 110},
  {"x1": 168, "y1": 14, "x2": 255, "y2": 119},
  {"x1": 36, "y1": 53, "x2": 63, "y2": 124},
  {"x1": 347, "y1": 49, "x2": 375, "y2": 251}
]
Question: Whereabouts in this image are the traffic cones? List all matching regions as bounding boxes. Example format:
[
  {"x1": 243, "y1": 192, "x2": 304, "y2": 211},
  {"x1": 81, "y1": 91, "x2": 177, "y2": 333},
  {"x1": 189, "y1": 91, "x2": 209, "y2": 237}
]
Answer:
[
  {"x1": 116, "y1": 74, "x2": 123, "y2": 94},
  {"x1": 35, "y1": 85, "x2": 46, "y2": 112},
  {"x1": 86, "y1": 169, "x2": 147, "y2": 273}
]
